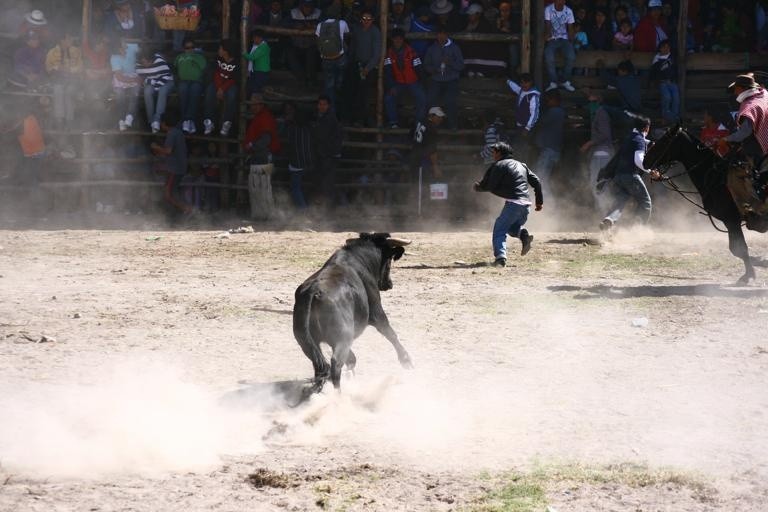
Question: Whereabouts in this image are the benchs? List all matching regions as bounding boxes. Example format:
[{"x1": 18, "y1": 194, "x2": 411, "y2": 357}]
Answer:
[{"x1": 0, "y1": 0, "x2": 767, "y2": 244}]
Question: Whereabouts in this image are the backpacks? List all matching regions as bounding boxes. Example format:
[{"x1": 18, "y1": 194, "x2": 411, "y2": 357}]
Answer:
[{"x1": 316, "y1": 17, "x2": 343, "y2": 58}]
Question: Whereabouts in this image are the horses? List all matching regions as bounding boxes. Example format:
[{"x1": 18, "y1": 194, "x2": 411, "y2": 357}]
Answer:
[{"x1": 642, "y1": 117, "x2": 768, "y2": 287}]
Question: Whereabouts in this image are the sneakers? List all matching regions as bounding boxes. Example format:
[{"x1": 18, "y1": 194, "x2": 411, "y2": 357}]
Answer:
[
  {"x1": 544, "y1": 81, "x2": 559, "y2": 93},
  {"x1": 520, "y1": 233, "x2": 534, "y2": 256},
  {"x1": 150, "y1": 121, "x2": 161, "y2": 134},
  {"x1": 219, "y1": 120, "x2": 233, "y2": 136},
  {"x1": 118, "y1": 118, "x2": 127, "y2": 132},
  {"x1": 123, "y1": 114, "x2": 134, "y2": 128},
  {"x1": 489, "y1": 258, "x2": 507, "y2": 268},
  {"x1": 201, "y1": 118, "x2": 215, "y2": 136},
  {"x1": 559, "y1": 80, "x2": 576, "y2": 93},
  {"x1": 598, "y1": 218, "x2": 613, "y2": 243},
  {"x1": 180, "y1": 119, "x2": 197, "y2": 136}
]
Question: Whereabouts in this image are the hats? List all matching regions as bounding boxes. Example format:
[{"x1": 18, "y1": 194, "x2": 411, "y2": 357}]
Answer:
[
  {"x1": 22, "y1": 9, "x2": 49, "y2": 27},
  {"x1": 464, "y1": 2, "x2": 484, "y2": 16},
  {"x1": 727, "y1": 74, "x2": 757, "y2": 92},
  {"x1": 427, "y1": 104, "x2": 447, "y2": 117},
  {"x1": 488, "y1": 141, "x2": 510, "y2": 154},
  {"x1": 391, "y1": 0, "x2": 405, "y2": 6},
  {"x1": 428, "y1": 0, "x2": 455, "y2": 16},
  {"x1": 244, "y1": 92, "x2": 272, "y2": 107},
  {"x1": 647, "y1": 0, "x2": 663, "y2": 9}
]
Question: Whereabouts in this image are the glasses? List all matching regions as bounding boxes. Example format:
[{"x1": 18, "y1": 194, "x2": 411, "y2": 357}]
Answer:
[
  {"x1": 362, "y1": 16, "x2": 373, "y2": 21},
  {"x1": 184, "y1": 46, "x2": 194, "y2": 50}
]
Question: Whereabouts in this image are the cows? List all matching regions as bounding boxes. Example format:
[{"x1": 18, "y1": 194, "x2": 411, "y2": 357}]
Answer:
[{"x1": 286, "y1": 231, "x2": 415, "y2": 409}]
